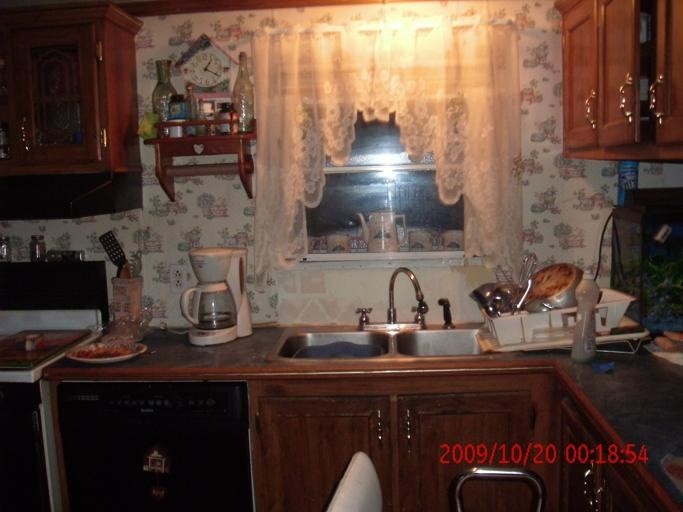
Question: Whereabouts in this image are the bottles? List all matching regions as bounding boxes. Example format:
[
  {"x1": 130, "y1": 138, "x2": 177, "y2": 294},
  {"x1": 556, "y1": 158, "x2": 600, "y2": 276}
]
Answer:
[
  {"x1": 150, "y1": 50, "x2": 252, "y2": 135},
  {"x1": 0, "y1": 236, "x2": 45, "y2": 263},
  {"x1": 618, "y1": 159, "x2": 639, "y2": 206}
]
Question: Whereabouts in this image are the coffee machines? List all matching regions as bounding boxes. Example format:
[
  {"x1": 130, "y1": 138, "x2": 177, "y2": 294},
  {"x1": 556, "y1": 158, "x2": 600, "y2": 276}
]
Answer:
[{"x1": 180, "y1": 246, "x2": 254, "y2": 347}]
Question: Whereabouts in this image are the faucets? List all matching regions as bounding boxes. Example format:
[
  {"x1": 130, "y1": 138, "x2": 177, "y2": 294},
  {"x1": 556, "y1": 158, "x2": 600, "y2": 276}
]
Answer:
[
  {"x1": 438, "y1": 298, "x2": 450, "y2": 328},
  {"x1": 387, "y1": 265, "x2": 429, "y2": 324}
]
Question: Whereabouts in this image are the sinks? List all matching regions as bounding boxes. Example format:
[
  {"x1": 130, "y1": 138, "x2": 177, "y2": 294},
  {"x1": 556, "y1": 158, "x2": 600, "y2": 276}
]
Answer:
[
  {"x1": 393, "y1": 322, "x2": 500, "y2": 356},
  {"x1": 269, "y1": 329, "x2": 395, "y2": 362}
]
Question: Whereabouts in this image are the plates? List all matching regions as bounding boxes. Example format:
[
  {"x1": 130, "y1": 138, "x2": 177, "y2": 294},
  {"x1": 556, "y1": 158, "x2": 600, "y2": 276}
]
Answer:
[{"x1": 66, "y1": 340, "x2": 148, "y2": 365}]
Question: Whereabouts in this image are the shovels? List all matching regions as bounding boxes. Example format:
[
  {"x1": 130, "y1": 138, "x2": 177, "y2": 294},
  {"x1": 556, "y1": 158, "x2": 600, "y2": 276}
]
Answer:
[{"x1": 99, "y1": 230, "x2": 126, "y2": 267}]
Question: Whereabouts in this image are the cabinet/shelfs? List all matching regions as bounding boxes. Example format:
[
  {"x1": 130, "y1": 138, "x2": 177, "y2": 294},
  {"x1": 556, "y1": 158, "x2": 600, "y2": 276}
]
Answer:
[
  {"x1": 252, "y1": 373, "x2": 555, "y2": 512},
  {"x1": 556, "y1": 0, "x2": 683, "y2": 162},
  {"x1": 555, "y1": 383, "x2": 672, "y2": 512}
]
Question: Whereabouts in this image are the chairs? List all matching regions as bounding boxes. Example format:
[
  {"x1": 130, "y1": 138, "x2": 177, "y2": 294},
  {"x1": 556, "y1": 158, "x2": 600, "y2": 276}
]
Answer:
[{"x1": 1, "y1": 6, "x2": 142, "y2": 178}]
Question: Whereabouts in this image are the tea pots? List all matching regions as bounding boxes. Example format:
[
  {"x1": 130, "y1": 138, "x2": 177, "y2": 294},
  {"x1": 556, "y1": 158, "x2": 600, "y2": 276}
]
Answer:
[{"x1": 355, "y1": 209, "x2": 406, "y2": 253}]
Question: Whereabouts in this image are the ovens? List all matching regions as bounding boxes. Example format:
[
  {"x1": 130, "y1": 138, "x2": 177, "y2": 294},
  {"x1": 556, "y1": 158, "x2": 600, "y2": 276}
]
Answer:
[{"x1": 53, "y1": 379, "x2": 255, "y2": 512}]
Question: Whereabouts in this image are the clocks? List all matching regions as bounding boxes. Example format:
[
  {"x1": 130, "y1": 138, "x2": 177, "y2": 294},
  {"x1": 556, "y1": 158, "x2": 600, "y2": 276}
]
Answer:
[{"x1": 175, "y1": 33, "x2": 237, "y2": 89}]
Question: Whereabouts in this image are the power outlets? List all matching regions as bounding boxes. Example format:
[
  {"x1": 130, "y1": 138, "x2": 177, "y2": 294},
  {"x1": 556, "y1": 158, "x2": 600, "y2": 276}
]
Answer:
[{"x1": 170, "y1": 264, "x2": 186, "y2": 292}]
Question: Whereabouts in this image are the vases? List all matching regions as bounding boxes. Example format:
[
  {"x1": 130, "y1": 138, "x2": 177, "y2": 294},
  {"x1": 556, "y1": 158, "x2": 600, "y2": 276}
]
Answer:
[{"x1": 152, "y1": 60, "x2": 177, "y2": 137}]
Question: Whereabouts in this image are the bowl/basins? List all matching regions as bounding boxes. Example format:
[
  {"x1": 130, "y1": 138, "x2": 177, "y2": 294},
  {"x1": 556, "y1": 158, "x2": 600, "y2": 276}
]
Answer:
[{"x1": 473, "y1": 263, "x2": 583, "y2": 318}]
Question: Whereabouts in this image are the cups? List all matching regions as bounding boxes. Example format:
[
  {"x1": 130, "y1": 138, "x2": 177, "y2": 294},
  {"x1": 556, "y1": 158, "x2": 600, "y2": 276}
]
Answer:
[
  {"x1": 408, "y1": 229, "x2": 462, "y2": 252},
  {"x1": 325, "y1": 234, "x2": 349, "y2": 253}
]
